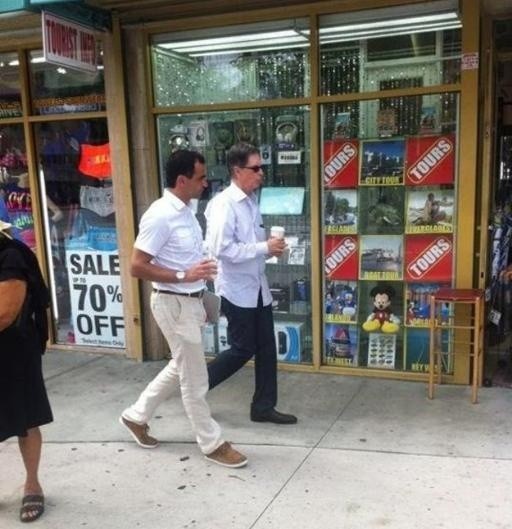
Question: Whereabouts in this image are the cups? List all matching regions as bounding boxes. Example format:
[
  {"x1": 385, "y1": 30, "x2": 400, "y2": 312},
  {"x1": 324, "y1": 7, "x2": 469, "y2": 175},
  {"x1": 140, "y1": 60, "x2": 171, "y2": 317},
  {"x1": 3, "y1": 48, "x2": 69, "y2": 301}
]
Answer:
[{"x1": 270, "y1": 226, "x2": 284, "y2": 239}]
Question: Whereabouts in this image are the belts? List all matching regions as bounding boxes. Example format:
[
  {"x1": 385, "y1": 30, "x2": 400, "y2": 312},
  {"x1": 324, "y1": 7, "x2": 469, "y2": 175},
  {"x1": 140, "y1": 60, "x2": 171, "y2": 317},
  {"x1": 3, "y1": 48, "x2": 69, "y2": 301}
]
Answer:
[{"x1": 152, "y1": 286, "x2": 204, "y2": 299}]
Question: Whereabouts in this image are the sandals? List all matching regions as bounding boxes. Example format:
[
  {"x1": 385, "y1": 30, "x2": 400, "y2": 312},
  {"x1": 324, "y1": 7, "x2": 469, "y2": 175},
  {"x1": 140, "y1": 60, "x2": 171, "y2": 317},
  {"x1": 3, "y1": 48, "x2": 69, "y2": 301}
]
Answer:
[{"x1": 19, "y1": 494, "x2": 44, "y2": 523}]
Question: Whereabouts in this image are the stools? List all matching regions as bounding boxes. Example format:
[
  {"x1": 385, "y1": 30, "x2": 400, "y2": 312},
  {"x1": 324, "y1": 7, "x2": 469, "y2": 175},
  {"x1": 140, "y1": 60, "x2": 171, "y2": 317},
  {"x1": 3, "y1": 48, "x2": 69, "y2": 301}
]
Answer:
[{"x1": 428, "y1": 288, "x2": 486, "y2": 404}]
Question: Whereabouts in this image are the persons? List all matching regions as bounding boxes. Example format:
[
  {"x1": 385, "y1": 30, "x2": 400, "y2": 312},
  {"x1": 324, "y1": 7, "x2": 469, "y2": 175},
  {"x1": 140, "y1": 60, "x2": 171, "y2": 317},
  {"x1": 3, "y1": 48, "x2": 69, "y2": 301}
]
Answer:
[
  {"x1": 1, "y1": 217, "x2": 55, "y2": 524},
  {"x1": 205, "y1": 141, "x2": 300, "y2": 426},
  {"x1": 117, "y1": 147, "x2": 250, "y2": 468},
  {"x1": 423, "y1": 193, "x2": 434, "y2": 224}
]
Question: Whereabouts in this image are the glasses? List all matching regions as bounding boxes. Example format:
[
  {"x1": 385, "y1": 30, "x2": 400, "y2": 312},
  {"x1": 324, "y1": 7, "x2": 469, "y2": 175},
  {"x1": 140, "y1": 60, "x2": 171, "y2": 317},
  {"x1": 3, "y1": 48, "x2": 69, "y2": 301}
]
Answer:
[{"x1": 241, "y1": 164, "x2": 266, "y2": 173}]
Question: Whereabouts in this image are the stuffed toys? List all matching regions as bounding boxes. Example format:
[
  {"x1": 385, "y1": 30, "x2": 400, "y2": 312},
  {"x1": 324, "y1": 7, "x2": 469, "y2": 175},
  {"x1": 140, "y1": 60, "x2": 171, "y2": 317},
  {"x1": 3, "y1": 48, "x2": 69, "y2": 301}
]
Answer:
[{"x1": 360, "y1": 285, "x2": 402, "y2": 334}]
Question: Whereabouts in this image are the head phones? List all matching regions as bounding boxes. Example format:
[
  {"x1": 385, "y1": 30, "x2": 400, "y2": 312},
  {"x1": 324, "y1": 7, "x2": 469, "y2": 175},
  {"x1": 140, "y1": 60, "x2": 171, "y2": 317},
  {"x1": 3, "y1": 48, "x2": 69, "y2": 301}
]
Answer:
[
  {"x1": 275, "y1": 122, "x2": 299, "y2": 144},
  {"x1": 196, "y1": 126, "x2": 205, "y2": 141}
]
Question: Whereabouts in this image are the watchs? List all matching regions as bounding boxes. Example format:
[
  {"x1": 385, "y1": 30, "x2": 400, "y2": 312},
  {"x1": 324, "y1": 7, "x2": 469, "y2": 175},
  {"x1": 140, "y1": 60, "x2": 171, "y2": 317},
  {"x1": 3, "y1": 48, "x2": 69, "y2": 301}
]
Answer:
[{"x1": 175, "y1": 270, "x2": 186, "y2": 281}]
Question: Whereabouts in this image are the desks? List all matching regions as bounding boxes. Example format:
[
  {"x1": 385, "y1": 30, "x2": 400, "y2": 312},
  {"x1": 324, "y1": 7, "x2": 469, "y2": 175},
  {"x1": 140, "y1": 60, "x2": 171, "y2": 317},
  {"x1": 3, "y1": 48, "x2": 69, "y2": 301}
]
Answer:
[{"x1": 65, "y1": 248, "x2": 126, "y2": 348}]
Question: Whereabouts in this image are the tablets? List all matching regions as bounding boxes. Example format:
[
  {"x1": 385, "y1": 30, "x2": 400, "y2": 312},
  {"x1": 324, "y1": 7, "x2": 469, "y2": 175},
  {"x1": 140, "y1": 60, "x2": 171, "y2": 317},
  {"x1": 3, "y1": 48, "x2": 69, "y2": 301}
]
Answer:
[{"x1": 202, "y1": 291, "x2": 221, "y2": 324}]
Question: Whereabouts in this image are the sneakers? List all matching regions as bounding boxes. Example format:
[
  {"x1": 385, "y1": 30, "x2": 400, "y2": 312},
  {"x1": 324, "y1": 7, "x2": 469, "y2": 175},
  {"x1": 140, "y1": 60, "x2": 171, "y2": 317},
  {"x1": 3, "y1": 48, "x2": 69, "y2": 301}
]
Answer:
[
  {"x1": 204, "y1": 441, "x2": 248, "y2": 468},
  {"x1": 119, "y1": 415, "x2": 159, "y2": 448}
]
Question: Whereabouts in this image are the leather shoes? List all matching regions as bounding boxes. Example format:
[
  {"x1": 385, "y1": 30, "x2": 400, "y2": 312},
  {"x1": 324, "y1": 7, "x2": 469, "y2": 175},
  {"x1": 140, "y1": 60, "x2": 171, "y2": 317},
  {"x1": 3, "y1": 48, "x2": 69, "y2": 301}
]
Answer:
[{"x1": 250, "y1": 402, "x2": 297, "y2": 424}]
